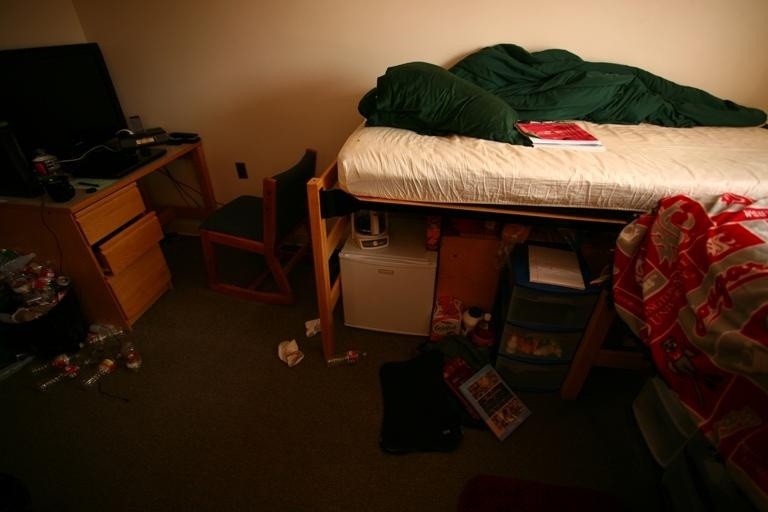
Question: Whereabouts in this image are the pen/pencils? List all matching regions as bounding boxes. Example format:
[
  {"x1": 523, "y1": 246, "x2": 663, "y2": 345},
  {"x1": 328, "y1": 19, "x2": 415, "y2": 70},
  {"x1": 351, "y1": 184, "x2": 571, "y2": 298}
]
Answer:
[{"x1": 77, "y1": 182, "x2": 99, "y2": 188}]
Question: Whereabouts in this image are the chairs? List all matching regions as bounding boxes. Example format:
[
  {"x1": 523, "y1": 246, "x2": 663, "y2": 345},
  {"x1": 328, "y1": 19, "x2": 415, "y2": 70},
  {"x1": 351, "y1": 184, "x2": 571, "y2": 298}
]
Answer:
[{"x1": 192, "y1": 147, "x2": 318, "y2": 306}]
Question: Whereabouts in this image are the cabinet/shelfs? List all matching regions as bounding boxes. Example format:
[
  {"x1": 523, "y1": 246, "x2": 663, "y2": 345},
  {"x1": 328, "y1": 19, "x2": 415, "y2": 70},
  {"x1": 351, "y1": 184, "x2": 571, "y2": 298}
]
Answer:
[{"x1": 493, "y1": 240, "x2": 601, "y2": 395}]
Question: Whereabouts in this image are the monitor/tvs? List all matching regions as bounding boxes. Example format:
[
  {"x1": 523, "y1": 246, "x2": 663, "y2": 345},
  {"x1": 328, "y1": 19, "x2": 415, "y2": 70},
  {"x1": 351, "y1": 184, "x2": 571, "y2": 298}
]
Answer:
[{"x1": 0, "y1": 42, "x2": 130, "y2": 176}]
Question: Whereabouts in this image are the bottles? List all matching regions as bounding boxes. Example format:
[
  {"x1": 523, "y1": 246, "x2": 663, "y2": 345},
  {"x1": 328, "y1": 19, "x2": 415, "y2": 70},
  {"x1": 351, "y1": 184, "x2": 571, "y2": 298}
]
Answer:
[
  {"x1": 39, "y1": 364, "x2": 82, "y2": 393},
  {"x1": 31, "y1": 353, "x2": 78, "y2": 376},
  {"x1": 31, "y1": 148, "x2": 76, "y2": 203},
  {"x1": 0, "y1": 247, "x2": 39, "y2": 299},
  {"x1": 78, "y1": 328, "x2": 123, "y2": 349},
  {"x1": 81, "y1": 352, "x2": 123, "y2": 392},
  {"x1": 0, "y1": 354, "x2": 36, "y2": 382},
  {"x1": 120, "y1": 342, "x2": 142, "y2": 374},
  {"x1": 469, "y1": 312, "x2": 498, "y2": 350},
  {"x1": 326, "y1": 350, "x2": 367, "y2": 368},
  {"x1": 461, "y1": 306, "x2": 484, "y2": 334}
]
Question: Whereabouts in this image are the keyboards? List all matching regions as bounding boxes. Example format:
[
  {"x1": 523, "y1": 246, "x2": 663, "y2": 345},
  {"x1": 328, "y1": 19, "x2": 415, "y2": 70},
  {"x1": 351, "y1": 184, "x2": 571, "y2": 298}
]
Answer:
[{"x1": 91, "y1": 147, "x2": 167, "y2": 179}]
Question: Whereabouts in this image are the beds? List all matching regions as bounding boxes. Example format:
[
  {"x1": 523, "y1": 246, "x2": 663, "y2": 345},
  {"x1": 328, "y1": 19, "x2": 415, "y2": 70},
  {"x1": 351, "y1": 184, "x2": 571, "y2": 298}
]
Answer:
[{"x1": 305, "y1": 117, "x2": 768, "y2": 366}]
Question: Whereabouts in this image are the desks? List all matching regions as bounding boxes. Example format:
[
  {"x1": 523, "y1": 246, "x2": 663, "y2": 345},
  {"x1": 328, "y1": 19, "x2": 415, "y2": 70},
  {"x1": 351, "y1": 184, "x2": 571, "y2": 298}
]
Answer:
[{"x1": 0, "y1": 136, "x2": 218, "y2": 333}]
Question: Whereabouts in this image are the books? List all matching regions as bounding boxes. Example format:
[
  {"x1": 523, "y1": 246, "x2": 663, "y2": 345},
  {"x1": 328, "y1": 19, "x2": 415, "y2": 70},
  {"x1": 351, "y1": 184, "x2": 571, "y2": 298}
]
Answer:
[
  {"x1": 512, "y1": 120, "x2": 602, "y2": 148},
  {"x1": 459, "y1": 364, "x2": 532, "y2": 442},
  {"x1": 528, "y1": 243, "x2": 586, "y2": 291}
]
